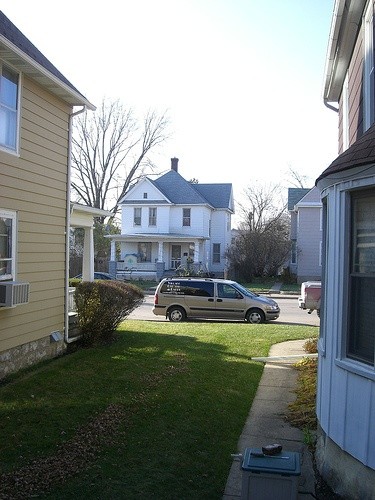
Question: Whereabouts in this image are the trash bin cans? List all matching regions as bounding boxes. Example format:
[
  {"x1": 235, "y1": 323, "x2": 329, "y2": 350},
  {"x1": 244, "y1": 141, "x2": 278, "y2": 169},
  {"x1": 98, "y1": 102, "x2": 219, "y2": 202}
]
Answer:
[{"x1": 237, "y1": 447, "x2": 304, "y2": 499}]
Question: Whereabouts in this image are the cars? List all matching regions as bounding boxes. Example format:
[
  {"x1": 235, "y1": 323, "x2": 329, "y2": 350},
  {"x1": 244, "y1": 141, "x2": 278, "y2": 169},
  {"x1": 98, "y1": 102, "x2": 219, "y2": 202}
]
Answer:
[{"x1": 73, "y1": 272, "x2": 118, "y2": 281}]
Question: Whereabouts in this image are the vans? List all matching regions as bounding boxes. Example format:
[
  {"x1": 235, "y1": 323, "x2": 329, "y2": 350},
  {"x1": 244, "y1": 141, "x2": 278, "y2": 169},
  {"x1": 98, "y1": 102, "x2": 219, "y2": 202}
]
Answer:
[{"x1": 152, "y1": 276, "x2": 281, "y2": 324}]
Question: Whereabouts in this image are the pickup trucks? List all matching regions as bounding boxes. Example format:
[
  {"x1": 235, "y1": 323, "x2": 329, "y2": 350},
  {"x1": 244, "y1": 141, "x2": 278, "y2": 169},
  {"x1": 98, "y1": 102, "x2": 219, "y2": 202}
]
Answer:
[{"x1": 297, "y1": 281, "x2": 322, "y2": 319}]
